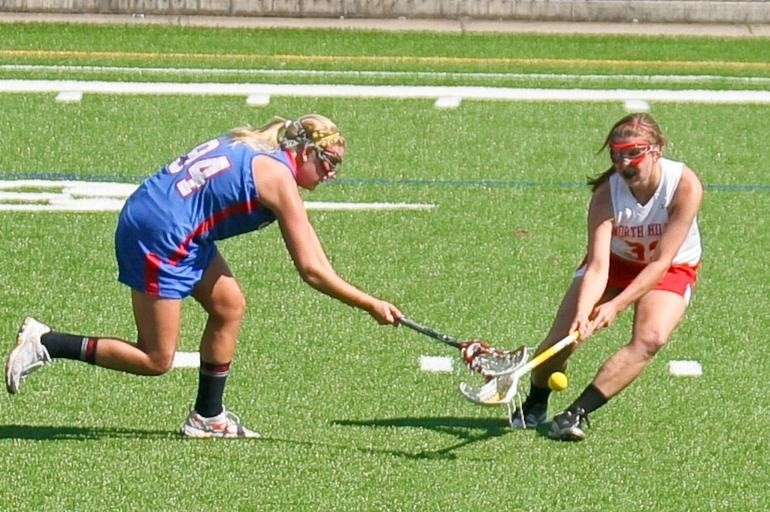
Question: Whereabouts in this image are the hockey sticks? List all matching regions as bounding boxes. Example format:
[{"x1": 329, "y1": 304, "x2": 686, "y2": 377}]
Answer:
[
  {"x1": 395, "y1": 317, "x2": 527, "y2": 376},
  {"x1": 458, "y1": 320, "x2": 595, "y2": 406}
]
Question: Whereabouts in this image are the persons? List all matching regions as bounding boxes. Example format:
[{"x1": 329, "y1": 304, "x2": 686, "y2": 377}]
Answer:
[
  {"x1": 4, "y1": 112, "x2": 407, "y2": 440},
  {"x1": 507, "y1": 110, "x2": 703, "y2": 442}
]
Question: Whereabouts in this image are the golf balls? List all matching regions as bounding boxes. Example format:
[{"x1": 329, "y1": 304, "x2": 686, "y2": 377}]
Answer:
[{"x1": 548, "y1": 371, "x2": 567, "y2": 391}]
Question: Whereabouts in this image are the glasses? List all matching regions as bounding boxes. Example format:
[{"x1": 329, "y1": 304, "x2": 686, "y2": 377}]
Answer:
[
  {"x1": 320, "y1": 150, "x2": 341, "y2": 170},
  {"x1": 607, "y1": 140, "x2": 650, "y2": 163}
]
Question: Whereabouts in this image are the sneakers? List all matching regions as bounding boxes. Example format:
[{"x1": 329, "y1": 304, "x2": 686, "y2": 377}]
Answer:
[
  {"x1": 181, "y1": 402, "x2": 261, "y2": 440},
  {"x1": 511, "y1": 387, "x2": 545, "y2": 431},
  {"x1": 550, "y1": 410, "x2": 591, "y2": 442},
  {"x1": 4, "y1": 313, "x2": 53, "y2": 396}
]
